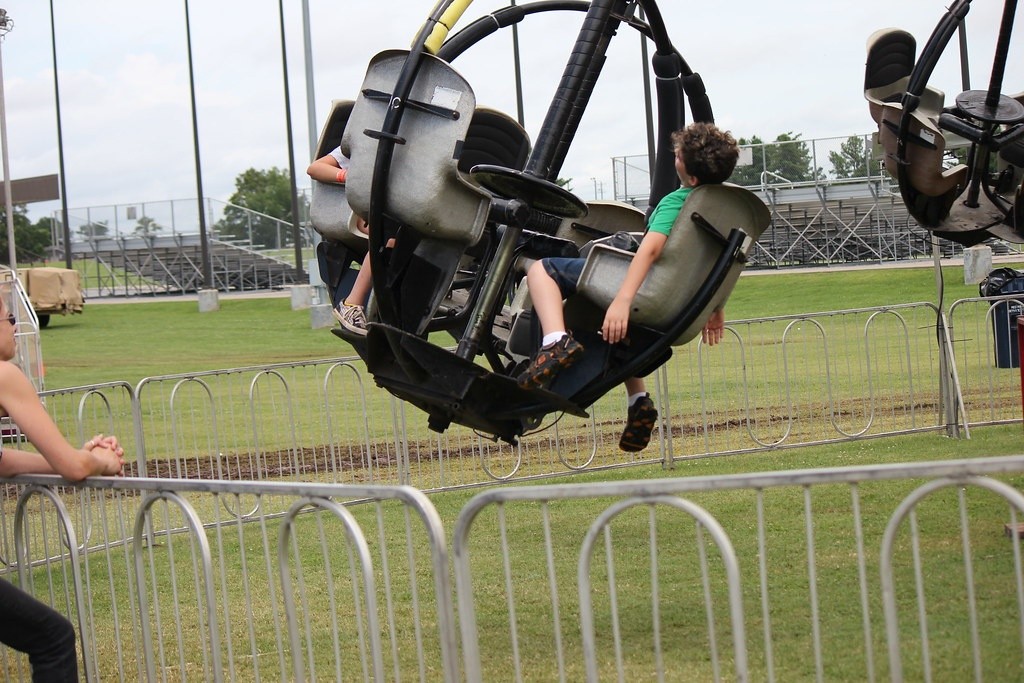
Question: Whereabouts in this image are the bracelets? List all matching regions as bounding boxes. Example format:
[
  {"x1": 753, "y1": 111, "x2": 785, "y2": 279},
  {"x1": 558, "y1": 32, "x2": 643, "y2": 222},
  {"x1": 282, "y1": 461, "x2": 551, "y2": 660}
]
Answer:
[{"x1": 337, "y1": 168, "x2": 347, "y2": 183}]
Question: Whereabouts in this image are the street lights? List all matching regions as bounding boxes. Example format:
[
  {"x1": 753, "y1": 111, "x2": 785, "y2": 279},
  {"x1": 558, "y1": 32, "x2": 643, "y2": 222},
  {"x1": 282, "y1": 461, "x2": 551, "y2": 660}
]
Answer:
[{"x1": 0, "y1": 7, "x2": 16, "y2": 275}]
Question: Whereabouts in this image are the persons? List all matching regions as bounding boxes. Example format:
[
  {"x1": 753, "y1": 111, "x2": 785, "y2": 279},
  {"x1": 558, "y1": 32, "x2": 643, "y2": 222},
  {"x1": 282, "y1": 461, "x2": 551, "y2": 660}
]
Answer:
[
  {"x1": 305, "y1": 144, "x2": 395, "y2": 337},
  {"x1": 1, "y1": 297, "x2": 126, "y2": 683},
  {"x1": 514, "y1": 121, "x2": 740, "y2": 453}
]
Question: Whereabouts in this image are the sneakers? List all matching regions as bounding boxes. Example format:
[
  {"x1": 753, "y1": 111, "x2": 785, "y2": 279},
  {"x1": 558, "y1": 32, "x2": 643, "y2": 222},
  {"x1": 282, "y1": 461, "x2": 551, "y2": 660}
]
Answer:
[
  {"x1": 517, "y1": 328, "x2": 585, "y2": 390},
  {"x1": 333, "y1": 299, "x2": 368, "y2": 336},
  {"x1": 619, "y1": 391, "x2": 658, "y2": 452}
]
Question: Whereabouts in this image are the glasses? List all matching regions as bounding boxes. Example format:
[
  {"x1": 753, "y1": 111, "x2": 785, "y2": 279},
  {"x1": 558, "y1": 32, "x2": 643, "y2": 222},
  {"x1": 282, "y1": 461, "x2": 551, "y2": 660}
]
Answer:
[{"x1": 0, "y1": 313, "x2": 16, "y2": 325}]
